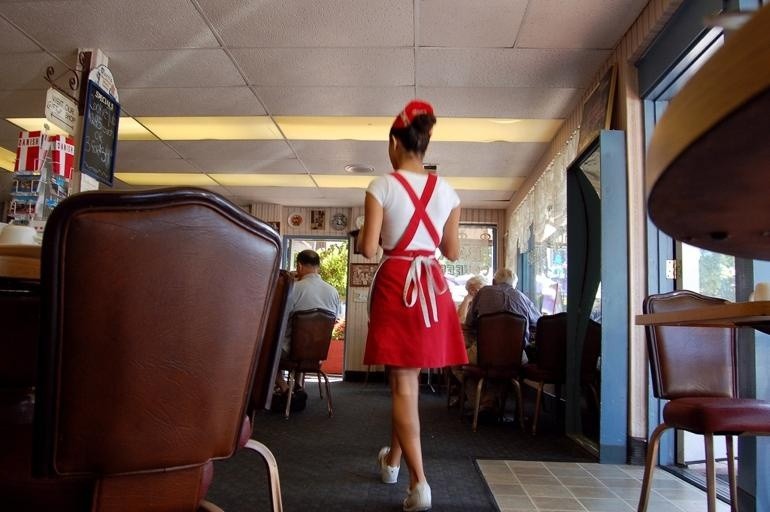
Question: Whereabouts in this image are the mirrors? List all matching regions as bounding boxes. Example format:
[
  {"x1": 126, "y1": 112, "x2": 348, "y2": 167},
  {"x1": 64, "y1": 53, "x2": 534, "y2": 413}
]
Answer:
[{"x1": 564, "y1": 129, "x2": 628, "y2": 461}]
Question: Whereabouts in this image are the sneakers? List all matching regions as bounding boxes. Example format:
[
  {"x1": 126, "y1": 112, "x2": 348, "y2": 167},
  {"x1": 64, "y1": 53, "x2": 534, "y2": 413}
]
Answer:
[
  {"x1": 463, "y1": 407, "x2": 494, "y2": 424},
  {"x1": 379, "y1": 446, "x2": 402, "y2": 484},
  {"x1": 403, "y1": 481, "x2": 432, "y2": 511}
]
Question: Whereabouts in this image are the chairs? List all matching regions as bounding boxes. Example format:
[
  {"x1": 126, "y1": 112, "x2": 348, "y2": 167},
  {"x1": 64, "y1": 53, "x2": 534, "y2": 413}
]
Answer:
[
  {"x1": 249, "y1": 265, "x2": 336, "y2": 419},
  {"x1": 0, "y1": 185, "x2": 291, "y2": 511},
  {"x1": 627, "y1": 284, "x2": 770, "y2": 512},
  {"x1": 581, "y1": 319, "x2": 600, "y2": 407},
  {"x1": 425, "y1": 311, "x2": 565, "y2": 437}
]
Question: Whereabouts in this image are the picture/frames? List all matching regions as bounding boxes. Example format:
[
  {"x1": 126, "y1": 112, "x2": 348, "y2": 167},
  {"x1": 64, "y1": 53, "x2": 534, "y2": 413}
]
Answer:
[{"x1": 348, "y1": 263, "x2": 380, "y2": 286}]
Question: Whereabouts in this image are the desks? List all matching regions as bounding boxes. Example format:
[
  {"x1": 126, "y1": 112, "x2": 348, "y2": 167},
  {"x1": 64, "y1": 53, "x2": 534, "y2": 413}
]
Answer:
[
  {"x1": 0, "y1": 252, "x2": 43, "y2": 285},
  {"x1": 631, "y1": 5, "x2": 770, "y2": 262},
  {"x1": 631, "y1": 298, "x2": 770, "y2": 334}
]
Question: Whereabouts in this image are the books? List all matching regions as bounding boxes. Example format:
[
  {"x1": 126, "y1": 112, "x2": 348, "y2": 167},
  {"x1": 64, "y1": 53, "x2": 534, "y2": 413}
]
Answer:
[{"x1": 9, "y1": 129, "x2": 75, "y2": 216}]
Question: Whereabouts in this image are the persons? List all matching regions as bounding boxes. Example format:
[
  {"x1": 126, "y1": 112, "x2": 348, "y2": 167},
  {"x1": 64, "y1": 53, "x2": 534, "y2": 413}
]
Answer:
[
  {"x1": 449, "y1": 275, "x2": 490, "y2": 416},
  {"x1": 354, "y1": 100, "x2": 471, "y2": 512},
  {"x1": 272, "y1": 249, "x2": 342, "y2": 415},
  {"x1": 465, "y1": 267, "x2": 543, "y2": 428}
]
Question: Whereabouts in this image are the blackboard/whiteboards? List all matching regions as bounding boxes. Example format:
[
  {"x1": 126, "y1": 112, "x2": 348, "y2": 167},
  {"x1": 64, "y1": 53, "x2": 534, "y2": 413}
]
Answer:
[{"x1": 79, "y1": 79, "x2": 121, "y2": 188}]
